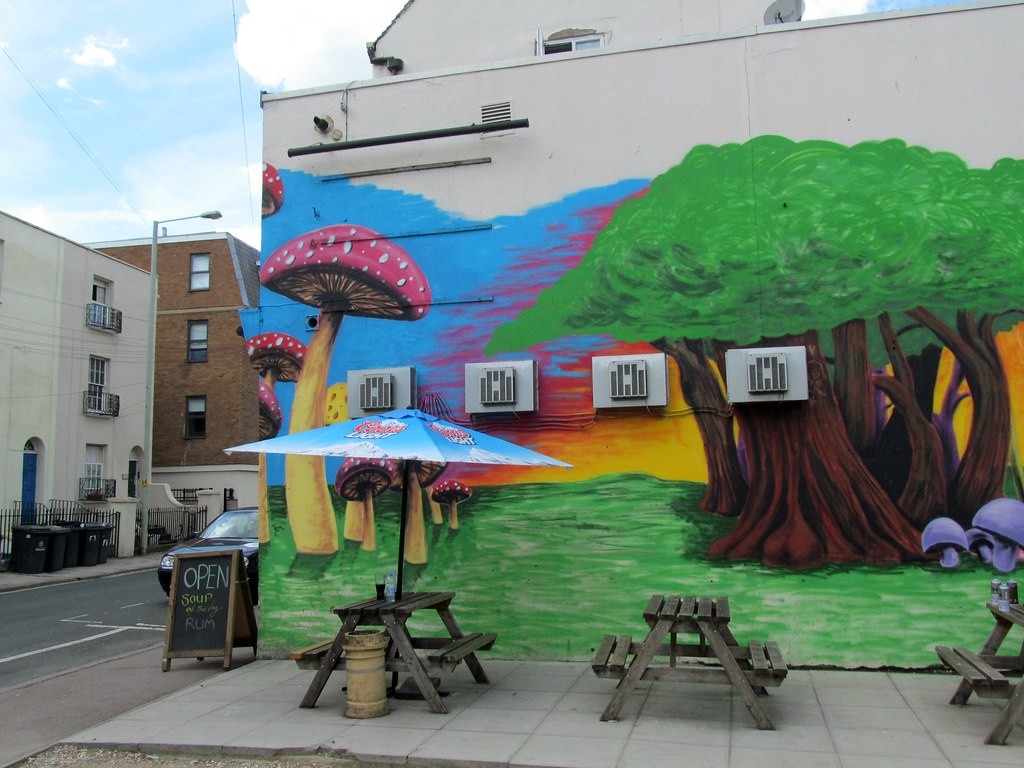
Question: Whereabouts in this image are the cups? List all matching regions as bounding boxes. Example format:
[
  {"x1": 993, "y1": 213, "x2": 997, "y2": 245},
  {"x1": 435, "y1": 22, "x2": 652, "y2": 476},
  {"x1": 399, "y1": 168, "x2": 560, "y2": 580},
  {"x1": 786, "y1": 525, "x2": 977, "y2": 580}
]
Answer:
[{"x1": 373, "y1": 574, "x2": 385, "y2": 601}]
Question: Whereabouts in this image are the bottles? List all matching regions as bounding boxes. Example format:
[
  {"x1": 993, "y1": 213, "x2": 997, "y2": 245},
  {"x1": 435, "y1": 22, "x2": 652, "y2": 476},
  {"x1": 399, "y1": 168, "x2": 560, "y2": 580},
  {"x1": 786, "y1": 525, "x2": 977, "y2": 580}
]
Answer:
[
  {"x1": 990, "y1": 579, "x2": 1001, "y2": 604},
  {"x1": 998, "y1": 584, "x2": 1010, "y2": 613},
  {"x1": 384, "y1": 570, "x2": 396, "y2": 604},
  {"x1": 1008, "y1": 579, "x2": 1018, "y2": 604}
]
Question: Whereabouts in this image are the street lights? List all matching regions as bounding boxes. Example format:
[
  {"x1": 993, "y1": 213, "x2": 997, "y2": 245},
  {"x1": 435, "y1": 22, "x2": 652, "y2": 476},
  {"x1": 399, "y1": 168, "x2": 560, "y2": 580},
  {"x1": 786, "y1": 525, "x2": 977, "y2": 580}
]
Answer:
[{"x1": 140, "y1": 210, "x2": 222, "y2": 555}]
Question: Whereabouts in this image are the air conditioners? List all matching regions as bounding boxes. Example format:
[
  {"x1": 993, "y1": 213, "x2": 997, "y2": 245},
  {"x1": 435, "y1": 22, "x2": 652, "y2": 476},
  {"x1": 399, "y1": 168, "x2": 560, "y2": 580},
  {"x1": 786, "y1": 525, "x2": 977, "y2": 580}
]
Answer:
[
  {"x1": 726, "y1": 345, "x2": 809, "y2": 404},
  {"x1": 592, "y1": 352, "x2": 670, "y2": 409},
  {"x1": 464, "y1": 360, "x2": 540, "y2": 414},
  {"x1": 346, "y1": 367, "x2": 416, "y2": 421}
]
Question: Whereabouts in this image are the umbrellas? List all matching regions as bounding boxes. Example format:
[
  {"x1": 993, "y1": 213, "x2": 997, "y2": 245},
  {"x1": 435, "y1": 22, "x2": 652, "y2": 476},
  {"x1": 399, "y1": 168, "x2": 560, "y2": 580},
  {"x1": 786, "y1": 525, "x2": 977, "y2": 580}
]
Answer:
[{"x1": 223, "y1": 405, "x2": 575, "y2": 700}]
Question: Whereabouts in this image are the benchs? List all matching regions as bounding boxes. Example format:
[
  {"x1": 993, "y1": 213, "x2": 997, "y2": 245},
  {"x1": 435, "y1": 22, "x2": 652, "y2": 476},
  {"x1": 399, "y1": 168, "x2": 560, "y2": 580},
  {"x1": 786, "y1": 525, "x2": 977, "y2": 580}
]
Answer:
[
  {"x1": 936, "y1": 645, "x2": 1024, "y2": 746},
  {"x1": 291, "y1": 625, "x2": 350, "y2": 710},
  {"x1": 729, "y1": 641, "x2": 789, "y2": 730},
  {"x1": 418, "y1": 632, "x2": 498, "y2": 714},
  {"x1": 591, "y1": 634, "x2": 651, "y2": 722}
]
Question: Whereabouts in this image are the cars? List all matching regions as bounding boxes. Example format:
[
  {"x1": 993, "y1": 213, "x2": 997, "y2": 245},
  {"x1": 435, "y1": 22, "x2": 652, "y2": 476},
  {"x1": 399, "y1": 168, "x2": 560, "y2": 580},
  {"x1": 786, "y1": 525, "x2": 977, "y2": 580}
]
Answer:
[{"x1": 157, "y1": 506, "x2": 258, "y2": 600}]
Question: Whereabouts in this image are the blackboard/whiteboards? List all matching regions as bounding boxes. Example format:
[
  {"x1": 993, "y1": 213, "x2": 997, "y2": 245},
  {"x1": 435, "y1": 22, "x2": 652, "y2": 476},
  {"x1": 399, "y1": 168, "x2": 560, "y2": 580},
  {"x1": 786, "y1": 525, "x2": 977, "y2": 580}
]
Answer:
[{"x1": 163, "y1": 549, "x2": 258, "y2": 660}]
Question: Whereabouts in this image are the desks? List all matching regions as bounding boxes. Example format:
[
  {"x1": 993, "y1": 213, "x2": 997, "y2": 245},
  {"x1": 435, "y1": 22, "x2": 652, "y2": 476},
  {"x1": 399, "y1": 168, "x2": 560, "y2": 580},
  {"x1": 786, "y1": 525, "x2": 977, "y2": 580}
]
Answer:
[
  {"x1": 331, "y1": 591, "x2": 456, "y2": 696},
  {"x1": 985, "y1": 604, "x2": 1024, "y2": 645},
  {"x1": 642, "y1": 594, "x2": 732, "y2": 686}
]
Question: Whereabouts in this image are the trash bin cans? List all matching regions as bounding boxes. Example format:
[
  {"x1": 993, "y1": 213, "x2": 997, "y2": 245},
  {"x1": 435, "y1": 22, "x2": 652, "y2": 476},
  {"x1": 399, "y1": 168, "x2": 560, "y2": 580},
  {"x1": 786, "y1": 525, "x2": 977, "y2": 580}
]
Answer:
[
  {"x1": 10, "y1": 524, "x2": 51, "y2": 575},
  {"x1": 97, "y1": 524, "x2": 113, "y2": 565},
  {"x1": 79, "y1": 523, "x2": 105, "y2": 567},
  {"x1": 59, "y1": 522, "x2": 85, "y2": 568},
  {"x1": 340, "y1": 628, "x2": 391, "y2": 719},
  {"x1": 45, "y1": 525, "x2": 70, "y2": 572}
]
